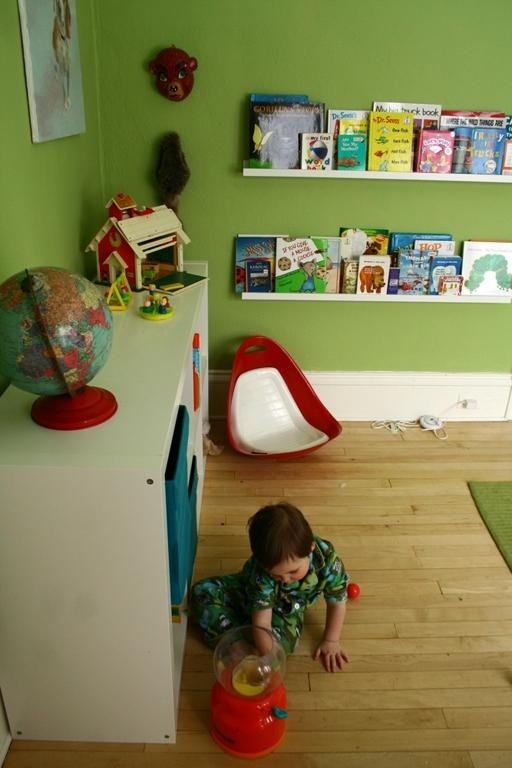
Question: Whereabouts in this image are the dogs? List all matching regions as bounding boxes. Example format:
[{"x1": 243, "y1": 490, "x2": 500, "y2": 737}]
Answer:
[{"x1": 53, "y1": 0, "x2": 74, "y2": 110}]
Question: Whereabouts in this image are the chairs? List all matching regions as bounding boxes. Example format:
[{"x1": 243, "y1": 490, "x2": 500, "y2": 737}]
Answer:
[{"x1": 225, "y1": 335, "x2": 344, "y2": 460}]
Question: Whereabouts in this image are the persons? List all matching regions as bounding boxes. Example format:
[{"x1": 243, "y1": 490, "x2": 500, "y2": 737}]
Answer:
[{"x1": 188, "y1": 499, "x2": 349, "y2": 674}]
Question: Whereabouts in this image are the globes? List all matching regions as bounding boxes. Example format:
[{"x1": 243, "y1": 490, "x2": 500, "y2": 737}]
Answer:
[{"x1": 0, "y1": 267, "x2": 118, "y2": 431}]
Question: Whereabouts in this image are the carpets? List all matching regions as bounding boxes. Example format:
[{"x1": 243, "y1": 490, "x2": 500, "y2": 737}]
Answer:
[{"x1": 467, "y1": 479, "x2": 511, "y2": 575}]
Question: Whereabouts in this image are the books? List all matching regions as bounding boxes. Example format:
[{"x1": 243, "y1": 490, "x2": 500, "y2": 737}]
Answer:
[
  {"x1": 244, "y1": 91, "x2": 512, "y2": 176},
  {"x1": 232, "y1": 224, "x2": 512, "y2": 296}
]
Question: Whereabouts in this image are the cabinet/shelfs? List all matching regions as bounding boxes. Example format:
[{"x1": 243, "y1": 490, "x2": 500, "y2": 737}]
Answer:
[
  {"x1": 2, "y1": 259, "x2": 213, "y2": 753},
  {"x1": 233, "y1": 166, "x2": 511, "y2": 309}
]
